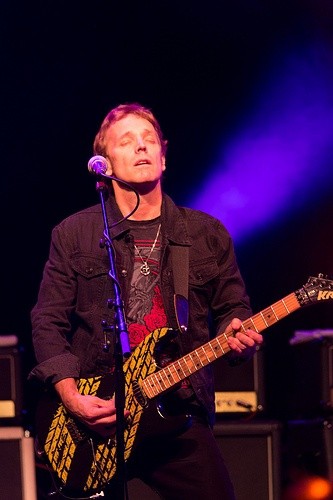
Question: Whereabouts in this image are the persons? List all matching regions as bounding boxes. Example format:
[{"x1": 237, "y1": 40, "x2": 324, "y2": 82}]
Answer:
[{"x1": 28, "y1": 105, "x2": 263, "y2": 500}]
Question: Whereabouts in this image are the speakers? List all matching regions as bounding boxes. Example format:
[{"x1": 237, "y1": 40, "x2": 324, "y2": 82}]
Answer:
[{"x1": 123, "y1": 424, "x2": 278, "y2": 500}]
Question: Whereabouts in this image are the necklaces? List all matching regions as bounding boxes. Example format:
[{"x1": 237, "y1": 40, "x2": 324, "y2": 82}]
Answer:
[{"x1": 132, "y1": 224, "x2": 160, "y2": 276}]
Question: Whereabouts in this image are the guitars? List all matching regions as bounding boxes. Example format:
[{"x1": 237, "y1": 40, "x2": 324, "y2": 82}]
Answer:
[{"x1": 34, "y1": 273, "x2": 333, "y2": 500}]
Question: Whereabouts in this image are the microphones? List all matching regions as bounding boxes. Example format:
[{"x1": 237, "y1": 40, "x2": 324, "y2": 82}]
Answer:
[{"x1": 87, "y1": 155, "x2": 108, "y2": 176}]
